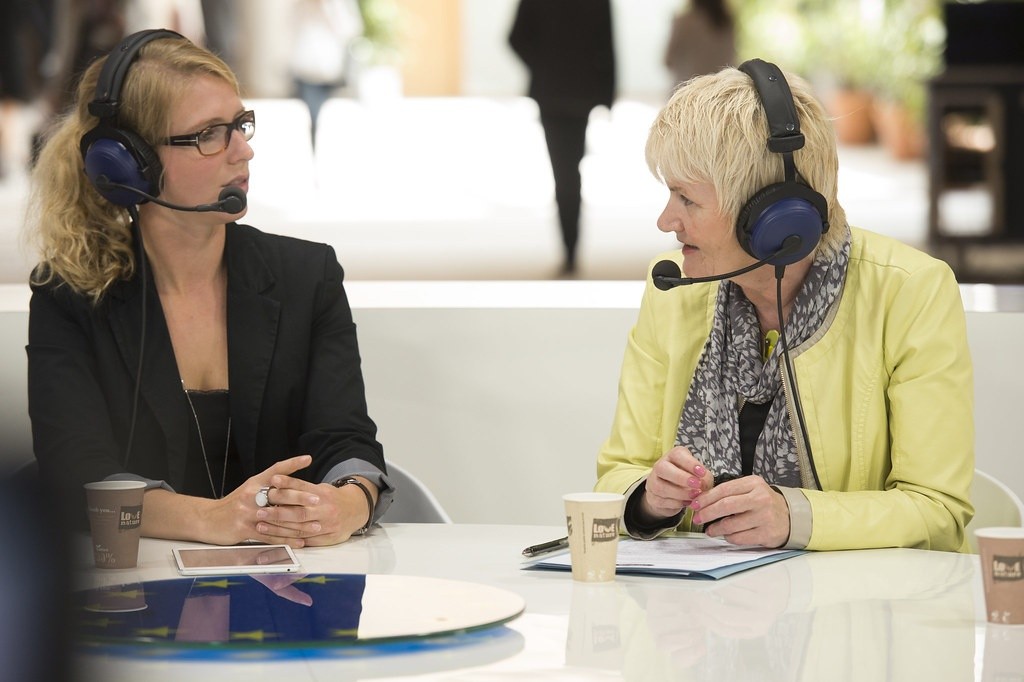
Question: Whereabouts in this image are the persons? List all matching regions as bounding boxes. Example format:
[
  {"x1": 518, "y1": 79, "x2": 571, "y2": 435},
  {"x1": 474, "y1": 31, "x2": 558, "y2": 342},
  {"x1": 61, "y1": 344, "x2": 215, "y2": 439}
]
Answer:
[
  {"x1": 25, "y1": 30, "x2": 397, "y2": 551},
  {"x1": 593, "y1": 59, "x2": 976, "y2": 555},
  {"x1": 667, "y1": 1, "x2": 740, "y2": 100},
  {"x1": 505, "y1": 1, "x2": 617, "y2": 275},
  {"x1": 73, "y1": 576, "x2": 366, "y2": 646},
  {"x1": 258, "y1": 548, "x2": 293, "y2": 565}
]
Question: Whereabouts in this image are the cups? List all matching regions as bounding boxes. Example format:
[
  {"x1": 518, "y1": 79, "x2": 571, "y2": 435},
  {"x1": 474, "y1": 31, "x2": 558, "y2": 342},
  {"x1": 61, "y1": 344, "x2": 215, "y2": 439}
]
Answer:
[
  {"x1": 559, "y1": 490, "x2": 624, "y2": 583},
  {"x1": 83, "y1": 480, "x2": 148, "y2": 569}
]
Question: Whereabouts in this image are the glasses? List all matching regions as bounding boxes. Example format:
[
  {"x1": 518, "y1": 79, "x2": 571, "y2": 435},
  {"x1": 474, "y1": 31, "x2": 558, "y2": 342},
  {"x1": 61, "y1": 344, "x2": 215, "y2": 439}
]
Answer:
[{"x1": 155, "y1": 110, "x2": 256, "y2": 156}]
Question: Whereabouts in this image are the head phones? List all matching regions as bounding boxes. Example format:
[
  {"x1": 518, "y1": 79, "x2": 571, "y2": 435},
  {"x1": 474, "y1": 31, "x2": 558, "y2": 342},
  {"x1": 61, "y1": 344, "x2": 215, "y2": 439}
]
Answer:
[
  {"x1": 736, "y1": 58, "x2": 830, "y2": 266},
  {"x1": 80, "y1": 29, "x2": 193, "y2": 207}
]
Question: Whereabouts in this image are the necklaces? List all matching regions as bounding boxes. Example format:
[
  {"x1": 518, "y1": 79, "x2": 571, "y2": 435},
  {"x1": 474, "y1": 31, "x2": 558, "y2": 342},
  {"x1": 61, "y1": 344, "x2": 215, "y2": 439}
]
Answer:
[
  {"x1": 756, "y1": 314, "x2": 789, "y2": 366},
  {"x1": 175, "y1": 363, "x2": 233, "y2": 500}
]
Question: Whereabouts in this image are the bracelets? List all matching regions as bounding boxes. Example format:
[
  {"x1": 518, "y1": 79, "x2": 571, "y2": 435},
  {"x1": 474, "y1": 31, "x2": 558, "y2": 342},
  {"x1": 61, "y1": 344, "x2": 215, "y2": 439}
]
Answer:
[{"x1": 332, "y1": 477, "x2": 375, "y2": 536}]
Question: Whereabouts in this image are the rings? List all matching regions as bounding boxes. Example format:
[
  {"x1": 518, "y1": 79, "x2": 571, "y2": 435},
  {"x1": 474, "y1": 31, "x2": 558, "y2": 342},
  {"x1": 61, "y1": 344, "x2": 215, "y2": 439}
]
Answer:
[{"x1": 254, "y1": 484, "x2": 278, "y2": 508}]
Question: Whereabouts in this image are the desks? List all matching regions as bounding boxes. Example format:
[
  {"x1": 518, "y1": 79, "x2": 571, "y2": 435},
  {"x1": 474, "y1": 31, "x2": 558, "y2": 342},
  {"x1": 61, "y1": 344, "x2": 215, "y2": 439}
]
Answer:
[{"x1": 63, "y1": 520, "x2": 1022, "y2": 682}]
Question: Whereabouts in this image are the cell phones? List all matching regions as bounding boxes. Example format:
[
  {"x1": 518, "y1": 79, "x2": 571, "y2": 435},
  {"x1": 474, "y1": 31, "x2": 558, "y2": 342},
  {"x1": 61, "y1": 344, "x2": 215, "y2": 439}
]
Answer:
[{"x1": 171, "y1": 544, "x2": 300, "y2": 576}]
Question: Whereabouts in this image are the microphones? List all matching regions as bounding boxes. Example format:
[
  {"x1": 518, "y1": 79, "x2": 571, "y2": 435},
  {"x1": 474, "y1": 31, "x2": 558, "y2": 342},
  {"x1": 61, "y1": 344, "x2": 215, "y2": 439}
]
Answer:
[
  {"x1": 653, "y1": 235, "x2": 802, "y2": 332},
  {"x1": 96, "y1": 173, "x2": 247, "y2": 214}
]
